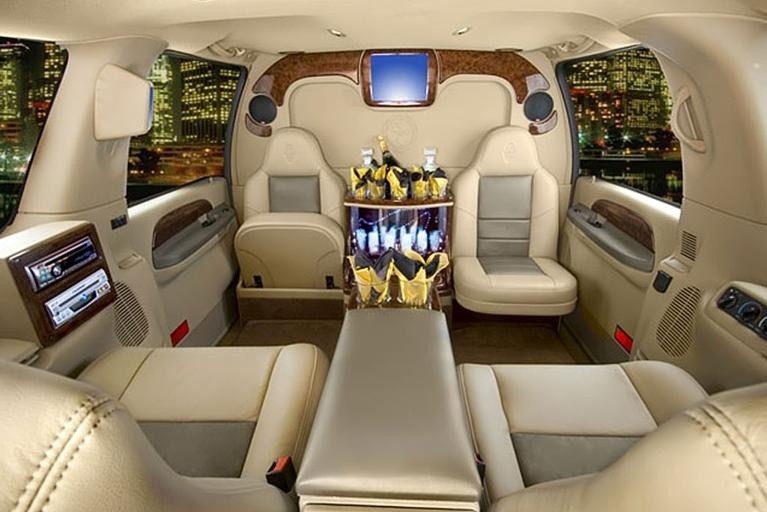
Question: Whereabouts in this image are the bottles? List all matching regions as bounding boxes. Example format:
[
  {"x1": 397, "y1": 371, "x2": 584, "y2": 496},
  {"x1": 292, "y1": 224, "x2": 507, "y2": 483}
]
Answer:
[{"x1": 377, "y1": 135, "x2": 401, "y2": 166}]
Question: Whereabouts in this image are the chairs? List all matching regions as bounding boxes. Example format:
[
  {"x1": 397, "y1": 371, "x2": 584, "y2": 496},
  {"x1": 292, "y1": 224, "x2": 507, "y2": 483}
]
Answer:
[
  {"x1": 1, "y1": 345, "x2": 330, "y2": 510},
  {"x1": 457, "y1": 361, "x2": 767, "y2": 511},
  {"x1": 447, "y1": 123, "x2": 578, "y2": 335},
  {"x1": 235, "y1": 128, "x2": 347, "y2": 325}
]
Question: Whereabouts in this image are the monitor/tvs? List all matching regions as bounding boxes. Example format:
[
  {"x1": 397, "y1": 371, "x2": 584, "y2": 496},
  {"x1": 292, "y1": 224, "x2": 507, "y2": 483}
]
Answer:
[{"x1": 359, "y1": 49, "x2": 440, "y2": 107}]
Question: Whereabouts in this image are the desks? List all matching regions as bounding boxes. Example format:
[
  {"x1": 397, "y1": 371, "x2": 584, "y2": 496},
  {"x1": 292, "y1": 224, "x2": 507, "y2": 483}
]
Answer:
[{"x1": 344, "y1": 191, "x2": 456, "y2": 330}]
